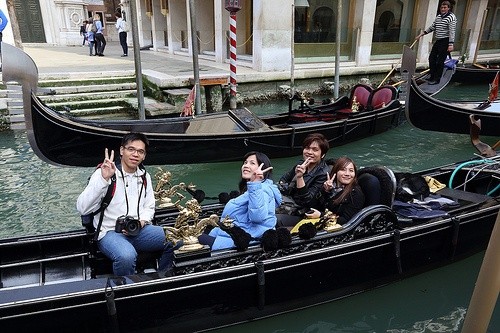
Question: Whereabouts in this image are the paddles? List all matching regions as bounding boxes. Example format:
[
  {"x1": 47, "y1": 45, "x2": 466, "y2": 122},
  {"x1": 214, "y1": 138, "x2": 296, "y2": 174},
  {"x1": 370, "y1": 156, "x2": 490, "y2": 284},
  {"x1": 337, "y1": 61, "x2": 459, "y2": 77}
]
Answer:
[
  {"x1": 393, "y1": 59, "x2": 451, "y2": 86},
  {"x1": 376, "y1": 39, "x2": 418, "y2": 89}
]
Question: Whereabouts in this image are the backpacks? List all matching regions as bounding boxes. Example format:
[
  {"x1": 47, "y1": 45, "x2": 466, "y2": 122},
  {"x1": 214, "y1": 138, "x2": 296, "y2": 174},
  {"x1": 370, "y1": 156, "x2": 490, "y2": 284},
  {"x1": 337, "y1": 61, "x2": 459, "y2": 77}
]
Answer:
[
  {"x1": 81, "y1": 164, "x2": 147, "y2": 232},
  {"x1": 122, "y1": 22, "x2": 130, "y2": 31},
  {"x1": 91, "y1": 22, "x2": 98, "y2": 33}
]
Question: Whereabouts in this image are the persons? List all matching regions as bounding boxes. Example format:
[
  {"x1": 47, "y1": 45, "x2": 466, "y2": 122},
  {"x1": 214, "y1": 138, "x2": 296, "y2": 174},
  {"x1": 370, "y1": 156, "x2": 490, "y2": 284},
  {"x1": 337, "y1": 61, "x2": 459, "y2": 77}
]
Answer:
[
  {"x1": 76, "y1": 133, "x2": 184, "y2": 278},
  {"x1": 93, "y1": 15, "x2": 106, "y2": 56},
  {"x1": 80, "y1": 18, "x2": 98, "y2": 56},
  {"x1": 115, "y1": 12, "x2": 128, "y2": 57},
  {"x1": 276, "y1": 156, "x2": 365, "y2": 231},
  {"x1": 198, "y1": 152, "x2": 282, "y2": 251},
  {"x1": 275, "y1": 134, "x2": 329, "y2": 215},
  {"x1": 0, "y1": 9, "x2": 8, "y2": 72},
  {"x1": 416, "y1": 1, "x2": 457, "y2": 85}
]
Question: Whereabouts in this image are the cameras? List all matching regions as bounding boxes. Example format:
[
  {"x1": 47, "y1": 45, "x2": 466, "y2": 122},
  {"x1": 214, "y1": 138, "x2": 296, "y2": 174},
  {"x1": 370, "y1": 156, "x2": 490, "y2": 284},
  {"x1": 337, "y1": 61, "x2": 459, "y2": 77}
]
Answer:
[{"x1": 115, "y1": 215, "x2": 138, "y2": 233}]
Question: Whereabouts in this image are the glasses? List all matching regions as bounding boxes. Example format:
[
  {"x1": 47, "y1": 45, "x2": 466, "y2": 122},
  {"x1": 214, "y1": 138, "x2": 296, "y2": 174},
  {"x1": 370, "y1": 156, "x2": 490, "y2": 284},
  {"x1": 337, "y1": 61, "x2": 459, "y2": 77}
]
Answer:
[{"x1": 126, "y1": 147, "x2": 144, "y2": 156}]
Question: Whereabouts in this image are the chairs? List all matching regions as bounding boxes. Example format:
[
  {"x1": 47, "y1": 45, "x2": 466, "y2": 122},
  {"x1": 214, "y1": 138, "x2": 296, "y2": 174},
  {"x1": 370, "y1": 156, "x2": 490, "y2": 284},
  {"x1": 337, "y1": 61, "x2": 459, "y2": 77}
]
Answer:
[
  {"x1": 369, "y1": 85, "x2": 398, "y2": 110},
  {"x1": 284, "y1": 89, "x2": 321, "y2": 122},
  {"x1": 335, "y1": 83, "x2": 373, "y2": 116},
  {"x1": 352, "y1": 163, "x2": 398, "y2": 208}
]
